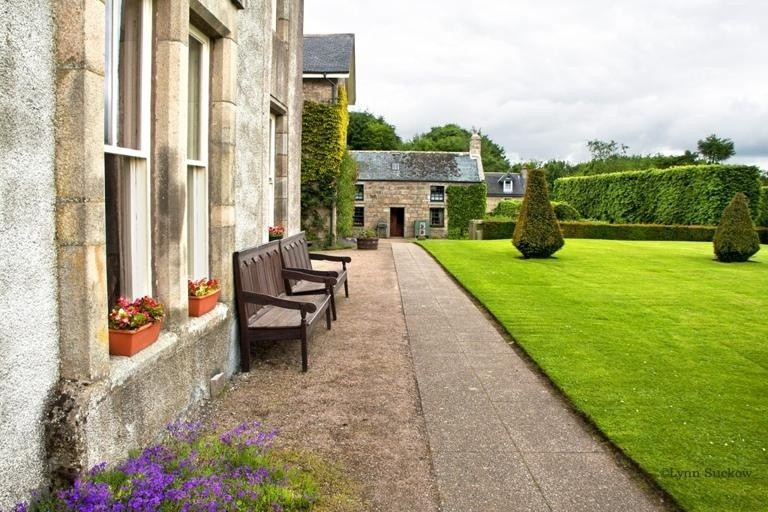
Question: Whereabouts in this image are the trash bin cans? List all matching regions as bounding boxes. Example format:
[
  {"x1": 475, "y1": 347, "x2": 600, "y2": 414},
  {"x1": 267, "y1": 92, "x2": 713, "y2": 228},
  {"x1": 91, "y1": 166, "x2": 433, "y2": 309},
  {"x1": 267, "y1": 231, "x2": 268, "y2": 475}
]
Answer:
[{"x1": 414, "y1": 219, "x2": 430, "y2": 239}]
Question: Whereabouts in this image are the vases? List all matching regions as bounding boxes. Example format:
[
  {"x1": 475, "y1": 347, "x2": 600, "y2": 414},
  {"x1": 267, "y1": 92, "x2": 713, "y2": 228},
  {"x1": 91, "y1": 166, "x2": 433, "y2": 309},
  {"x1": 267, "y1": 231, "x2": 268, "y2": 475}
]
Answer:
[
  {"x1": 188, "y1": 288, "x2": 221, "y2": 317},
  {"x1": 109, "y1": 315, "x2": 163, "y2": 357}
]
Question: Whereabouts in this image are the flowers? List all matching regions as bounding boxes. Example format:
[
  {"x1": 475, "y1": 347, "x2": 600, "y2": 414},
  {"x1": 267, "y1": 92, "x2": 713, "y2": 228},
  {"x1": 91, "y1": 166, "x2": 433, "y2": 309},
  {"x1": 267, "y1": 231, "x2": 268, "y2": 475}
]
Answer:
[
  {"x1": 107, "y1": 293, "x2": 166, "y2": 330},
  {"x1": 268, "y1": 225, "x2": 285, "y2": 242},
  {"x1": 187, "y1": 276, "x2": 223, "y2": 298}
]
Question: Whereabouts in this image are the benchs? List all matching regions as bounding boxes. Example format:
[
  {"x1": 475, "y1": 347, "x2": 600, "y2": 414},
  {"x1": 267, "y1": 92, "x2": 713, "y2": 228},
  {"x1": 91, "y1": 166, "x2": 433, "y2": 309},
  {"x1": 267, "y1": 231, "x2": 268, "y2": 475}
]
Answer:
[
  {"x1": 280, "y1": 230, "x2": 351, "y2": 321},
  {"x1": 231, "y1": 239, "x2": 337, "y2": 373}
]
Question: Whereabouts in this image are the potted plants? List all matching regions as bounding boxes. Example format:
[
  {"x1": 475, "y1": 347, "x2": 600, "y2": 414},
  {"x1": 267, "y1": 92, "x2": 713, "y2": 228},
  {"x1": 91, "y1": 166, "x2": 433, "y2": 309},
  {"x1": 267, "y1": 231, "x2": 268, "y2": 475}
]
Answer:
[{"x1": 356, "y1": 225, "x2": 380, "y2": 250}]
